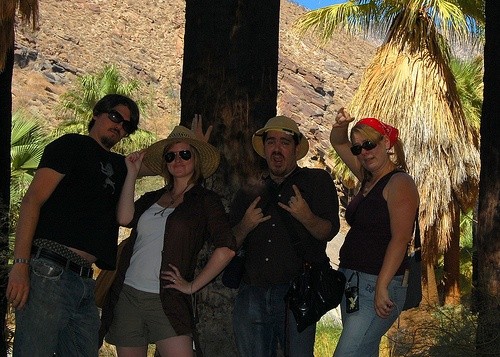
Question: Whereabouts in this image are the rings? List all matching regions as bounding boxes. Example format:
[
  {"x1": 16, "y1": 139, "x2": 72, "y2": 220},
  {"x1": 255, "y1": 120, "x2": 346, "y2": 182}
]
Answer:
[{"x1": 173, "y1": 279, "x2": 175, "y2": 282}]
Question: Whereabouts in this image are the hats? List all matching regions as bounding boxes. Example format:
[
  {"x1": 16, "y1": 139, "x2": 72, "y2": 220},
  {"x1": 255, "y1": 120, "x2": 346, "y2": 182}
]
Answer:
[
  {"x1": 138, "y1": 126, "x2": 220, "y2": 178},
  {"x1": 252, "y1": 115, "x2": 309, "y2": 160}
]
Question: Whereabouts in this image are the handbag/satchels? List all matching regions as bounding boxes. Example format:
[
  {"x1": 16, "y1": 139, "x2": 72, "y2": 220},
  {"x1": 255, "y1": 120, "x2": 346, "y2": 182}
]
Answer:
[
  {"x1": 223, "y1": 256, "x2": 243, "y2": 289},
  {"x1": 284, "y1": 262, "x2": 346, "y2": 333},
  {"x1": 94, "y1": 238, "x2": 130, "y2": 306},
  {"x1": 345, "y1": 272, "x2": 359, "y2": 313},
  {"x1": 402, "y1": 219, "x2": 422, "y2": 311}
]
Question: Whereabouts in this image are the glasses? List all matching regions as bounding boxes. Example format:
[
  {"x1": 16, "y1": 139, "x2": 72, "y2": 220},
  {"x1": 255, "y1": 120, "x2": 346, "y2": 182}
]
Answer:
[
  {"x1": 165, "y1": 150, "x2": 192, "y2": 163},
  {"x1": 105, "y1": 108, "x2": 138, "y2": 136},
  {"x1": 350, "y1": 136, "x2": 383, "y2": 155}
]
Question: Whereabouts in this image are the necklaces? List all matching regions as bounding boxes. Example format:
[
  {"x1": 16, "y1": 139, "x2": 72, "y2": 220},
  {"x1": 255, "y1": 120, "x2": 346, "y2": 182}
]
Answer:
[{"x1": 153, "y1": 181, "x2": 193, "y2": 217}]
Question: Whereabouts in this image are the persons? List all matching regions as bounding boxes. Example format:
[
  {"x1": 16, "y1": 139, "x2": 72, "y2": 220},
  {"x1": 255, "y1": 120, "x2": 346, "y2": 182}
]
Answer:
[
  {"x1": 99, "y1": 126, "x2": 238, "y2": 357},
  {"x1": 5, "y1": 93, "x2": 213, "y2": 357},
  {"x1": 230, "y1": 115, "x2": 341, "y2": 356},
  {"x1": 330, "y1": 106, "x2": 421, "y2": 357}
]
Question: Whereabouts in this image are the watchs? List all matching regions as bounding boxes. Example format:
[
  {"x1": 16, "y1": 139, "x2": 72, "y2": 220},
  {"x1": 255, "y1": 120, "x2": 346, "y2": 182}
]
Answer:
[{"x1": 12, "y1": 258, "x2": 28, "y2": 266}]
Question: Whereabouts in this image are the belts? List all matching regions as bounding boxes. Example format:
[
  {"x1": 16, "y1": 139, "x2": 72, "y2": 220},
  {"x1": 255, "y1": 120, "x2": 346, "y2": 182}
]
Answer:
[{"x1": 31, "y1": 244, "x2": 94, "y2": 277}]
